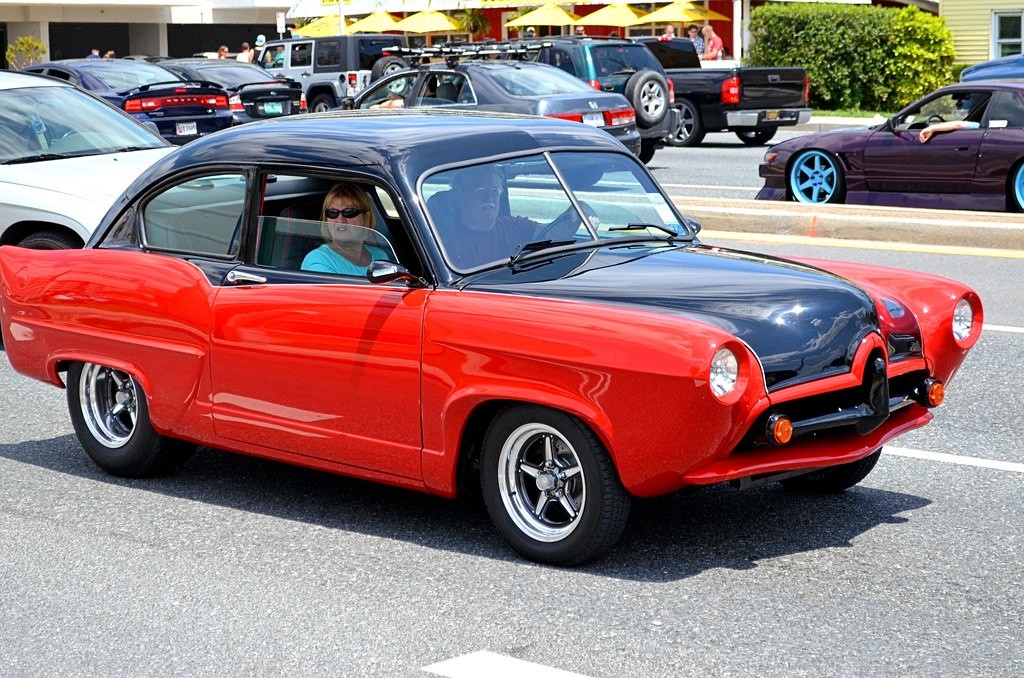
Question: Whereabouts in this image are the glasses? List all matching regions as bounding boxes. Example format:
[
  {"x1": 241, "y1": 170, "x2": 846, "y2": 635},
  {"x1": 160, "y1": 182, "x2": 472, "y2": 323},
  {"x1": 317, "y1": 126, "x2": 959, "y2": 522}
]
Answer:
[
  {"x1": 326, "y1": 208, "x2": 368, "y2": 218},
  {"x1": 469, "y1": 186, "x2": 504, "y2": 194}
]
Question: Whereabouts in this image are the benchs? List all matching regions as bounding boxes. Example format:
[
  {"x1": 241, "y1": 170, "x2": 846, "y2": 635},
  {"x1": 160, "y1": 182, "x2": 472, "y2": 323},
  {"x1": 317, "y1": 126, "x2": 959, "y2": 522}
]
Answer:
[{"x1": 146, "y1": 189, "x2": 329, "y2": 254}]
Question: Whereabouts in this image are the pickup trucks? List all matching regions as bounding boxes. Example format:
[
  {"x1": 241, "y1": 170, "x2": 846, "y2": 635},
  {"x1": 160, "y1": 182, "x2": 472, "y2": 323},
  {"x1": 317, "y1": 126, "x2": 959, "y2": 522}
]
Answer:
[{"x1": 628, "y1": 36, "x2": 813, "y2": 145}]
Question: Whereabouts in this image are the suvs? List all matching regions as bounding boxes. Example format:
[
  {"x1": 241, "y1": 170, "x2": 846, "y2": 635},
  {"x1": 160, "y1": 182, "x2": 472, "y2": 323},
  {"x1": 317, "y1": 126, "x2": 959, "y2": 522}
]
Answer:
[{"x1": 253, "y1": 31, "x2": 411, "y2": 113}]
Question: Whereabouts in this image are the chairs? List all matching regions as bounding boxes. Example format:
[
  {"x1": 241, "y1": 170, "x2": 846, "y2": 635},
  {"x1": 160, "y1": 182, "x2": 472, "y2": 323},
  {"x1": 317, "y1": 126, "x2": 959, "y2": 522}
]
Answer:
[
  {"x1": 427, "y1": 189, "x2": 461, "y2": 240},
  {"x1": 268, "y1": 191, "x2": 399, "y2": 271},
  {"x1": 435, "y1": 78, "x2": 456, "y2": 104}
]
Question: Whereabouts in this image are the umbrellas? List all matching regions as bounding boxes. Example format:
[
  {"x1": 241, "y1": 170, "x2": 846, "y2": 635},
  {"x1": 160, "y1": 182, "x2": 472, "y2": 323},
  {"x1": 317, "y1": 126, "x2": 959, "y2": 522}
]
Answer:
[
  {"x1": 291, "y1": 10, "x2": 465, "y2": 46},
  {"x1": 503, "y1": 0, "x2": 731, "y2": 38}
]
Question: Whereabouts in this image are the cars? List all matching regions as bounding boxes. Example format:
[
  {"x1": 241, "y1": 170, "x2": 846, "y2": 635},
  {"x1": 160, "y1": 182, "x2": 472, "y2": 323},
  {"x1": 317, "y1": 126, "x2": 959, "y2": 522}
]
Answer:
[
  {"x1": 754, "y1": 52, "x2": 1024, "y2": 210},
  {"x1": 0, "y1": 70, "x2": 181, "y2": 373},
  {"x1": 21, "y1": 58, "x2": 307, "y2": 144},
  {"x1": 0, "y1": 107, "x2": 985, "y2": 569},
  {"x1": 326, "y1": 34, "x2": 680, "y2": 164}
]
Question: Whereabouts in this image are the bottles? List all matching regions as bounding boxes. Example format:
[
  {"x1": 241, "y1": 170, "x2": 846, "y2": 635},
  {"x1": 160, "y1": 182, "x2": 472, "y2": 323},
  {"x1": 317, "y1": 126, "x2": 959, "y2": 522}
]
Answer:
[{"x1": 718, "y1": 49, "x2": 722, "y2": 61}]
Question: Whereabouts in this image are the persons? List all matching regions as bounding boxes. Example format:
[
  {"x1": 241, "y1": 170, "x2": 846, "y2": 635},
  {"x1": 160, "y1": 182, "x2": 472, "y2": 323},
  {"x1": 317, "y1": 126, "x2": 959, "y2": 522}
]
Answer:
[
  {"x1": 527, "y1": 27, "x2": 536, "y2": 37},
  {"x1": 236, "y1": 42, "x2": 251, "y2": 63},
  {"x1": 249, "y1": 34, "x2": 271, "y2": 63},
  {"x1": 918, "y1": 120, "x2": 981, "y2": 144},
  {"x1": 299, "y1": 183, "x2": 390, "y2": 278},
  {"x1": 575, "y1": 25, "x2": 585, "y2": 36},
  {"x1": 274, "y1": 46, "x2": 285, "y2": 63},
  {"x1": 698, "y1": 25, "x2": 723, "y2": 60},
  {"x1": 370, "y1": 74, "x2": 437, "y2": 108},
  {"x1": 218, "y1": 45, "x2": 229, "y2": 60},
  {"x1": 86, "y1": 49, "x2": 116, "y2": 58},
  {"x1": 687, "y1": 25, "x2": 704, "y2": 55},
  {"x1": 662, "y1": 25, "x2": 675, "y2": 37},
  {"x1": 439, "y1": 165, "x2": 601, "y2": 270}
]
